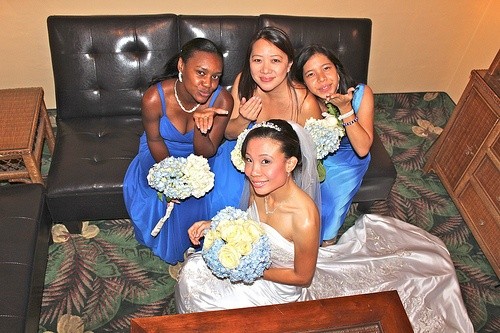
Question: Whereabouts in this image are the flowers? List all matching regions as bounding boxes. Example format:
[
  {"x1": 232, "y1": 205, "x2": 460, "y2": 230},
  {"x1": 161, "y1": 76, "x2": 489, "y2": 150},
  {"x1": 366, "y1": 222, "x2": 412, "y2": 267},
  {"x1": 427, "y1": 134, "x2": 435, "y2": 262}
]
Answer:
[
  {"x1": 231, "y1": 128, "x2": 248, "y2": 173},
  {"x1": 146, "y1": 153, "x2": 215, "y2": 203},
  {"x1": 304, "y1": 104, "x2": 346, "y2": 182},
  {"x1": 201, "y1": 205, "x2": 272, "y2": 285}
]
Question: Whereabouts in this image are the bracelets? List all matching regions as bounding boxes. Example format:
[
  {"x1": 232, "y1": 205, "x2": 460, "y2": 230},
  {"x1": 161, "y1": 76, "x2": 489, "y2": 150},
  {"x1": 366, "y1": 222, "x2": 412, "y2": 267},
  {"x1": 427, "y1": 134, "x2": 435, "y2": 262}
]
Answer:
[
  {"x1": 338, "y1": 109, "x2": 354, "y2": 120},
  {"x1": 343, "y1": 117, "x2": 358, "y2": 126}
]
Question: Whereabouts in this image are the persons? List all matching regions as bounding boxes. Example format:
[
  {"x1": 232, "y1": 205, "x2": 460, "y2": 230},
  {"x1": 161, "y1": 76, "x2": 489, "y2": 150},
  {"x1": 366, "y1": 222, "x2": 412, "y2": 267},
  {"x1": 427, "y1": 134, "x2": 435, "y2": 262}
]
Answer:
[
  {"x1": 123, "y1": 37, "x2": 234, "y2": 266},
  {"x1": 174, "y1": 117, "x2": 474, "y2": 333},
  {"x1": 293, "y1": 45, "x2": 373, "y2": 248},
  {"x1": 209, "y1": 26, "x2": 322, "y2": 224}
]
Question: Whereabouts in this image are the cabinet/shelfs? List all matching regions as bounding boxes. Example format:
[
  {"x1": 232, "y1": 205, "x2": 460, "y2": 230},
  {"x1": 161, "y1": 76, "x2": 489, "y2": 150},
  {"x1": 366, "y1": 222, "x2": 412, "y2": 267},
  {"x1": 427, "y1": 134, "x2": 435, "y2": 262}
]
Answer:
[{"x1": 424, "y1": 48, "x2": 500, "y2": 279}]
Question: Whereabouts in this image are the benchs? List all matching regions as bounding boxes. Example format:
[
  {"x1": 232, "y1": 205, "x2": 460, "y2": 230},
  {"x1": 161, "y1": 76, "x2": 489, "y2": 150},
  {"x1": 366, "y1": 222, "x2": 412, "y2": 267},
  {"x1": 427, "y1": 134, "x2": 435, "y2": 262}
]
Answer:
[{"x1": 0, "y1": 14, "x2": 397, "y2": 333}]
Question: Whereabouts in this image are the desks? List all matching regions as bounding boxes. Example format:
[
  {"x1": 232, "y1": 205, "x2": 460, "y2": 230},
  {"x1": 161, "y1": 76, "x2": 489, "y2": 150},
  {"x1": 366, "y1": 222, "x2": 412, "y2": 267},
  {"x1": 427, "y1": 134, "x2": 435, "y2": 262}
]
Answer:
[
  {"x1": 130, "y1": 290, "x2": 413, "y2": 333},
  {"x1": 0, "y1": 87, "x2": 56, "y2": 186}
]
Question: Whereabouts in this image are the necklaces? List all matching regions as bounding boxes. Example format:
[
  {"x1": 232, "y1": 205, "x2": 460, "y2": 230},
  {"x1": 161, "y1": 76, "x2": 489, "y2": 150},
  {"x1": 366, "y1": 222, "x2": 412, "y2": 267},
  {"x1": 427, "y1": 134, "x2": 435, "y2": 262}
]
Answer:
[
  {"x1": 173, "y1": 79, "x2": 201, "y2": 113},
  {"x1": 263, "y1": 196, "x2": 281, "y2": 215}
]
